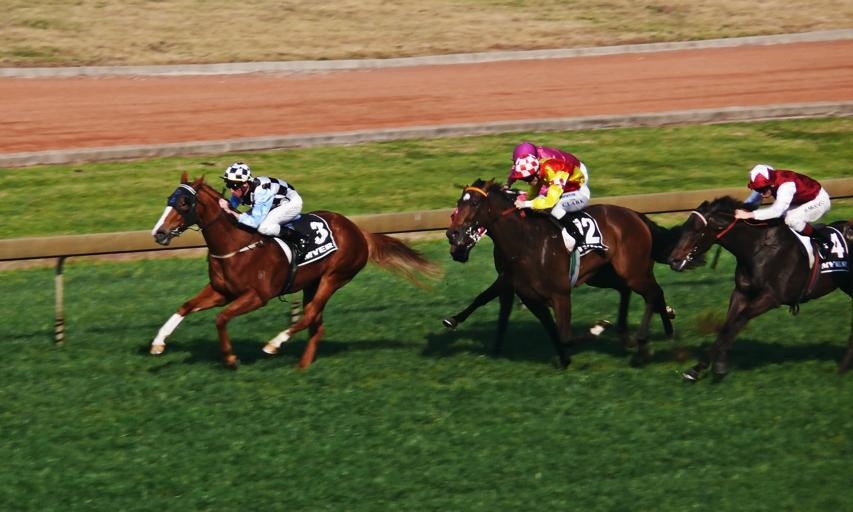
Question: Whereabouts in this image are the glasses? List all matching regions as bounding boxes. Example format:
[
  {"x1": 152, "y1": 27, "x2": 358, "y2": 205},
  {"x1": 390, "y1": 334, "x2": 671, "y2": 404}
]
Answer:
[{"x1": 226, "y1": 183, "x2": 241, "y2": 190}]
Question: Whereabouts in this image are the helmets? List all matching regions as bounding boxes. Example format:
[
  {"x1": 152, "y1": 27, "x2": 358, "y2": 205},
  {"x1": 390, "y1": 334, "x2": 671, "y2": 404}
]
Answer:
[
  {"x1": 219, "y1": 162, "x2": 251, "y2": 183},
  {"x1": 748, "y1": 164, "x2": 776, "y2": 189},
  {"x1": 510, "y1": 143, "x2": 540, "y2": 178}
]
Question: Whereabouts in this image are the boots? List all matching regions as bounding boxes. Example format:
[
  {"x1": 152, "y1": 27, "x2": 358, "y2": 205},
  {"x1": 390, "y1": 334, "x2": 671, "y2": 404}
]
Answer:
[
  {"x1": 277, "y1": 225, "x2": 310, "y2": 256},
  {"x1": 811, "y1": 229, "x2": 833, "y2": 255},
  {"x1": 559, "y1": 214, "x2": 584, "y2": 251}
]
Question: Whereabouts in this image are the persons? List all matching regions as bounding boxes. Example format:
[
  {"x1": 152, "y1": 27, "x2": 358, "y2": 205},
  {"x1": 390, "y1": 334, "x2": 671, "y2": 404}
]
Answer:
[
  {"x1": 502, "y1": 143, "x2": 591, "y2": 251},
  {"x1": 733, "y1": 164, "x2": 832, "y2": 259},
  {"x1": 218, "y1": 162, "x2": 309, "y2": 261}
]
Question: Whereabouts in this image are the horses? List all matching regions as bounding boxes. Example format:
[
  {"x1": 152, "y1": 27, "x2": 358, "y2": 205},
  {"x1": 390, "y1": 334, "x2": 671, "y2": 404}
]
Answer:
[
  {"x1": 148, "y1": 169, "x2": 448, "y2": 369},
  {"x1": 443, "y1": 176, "x2": 707, "y2": 372},
  {"x1": 664, "y1": 193, "x2": 853, "y2": 382}
]
